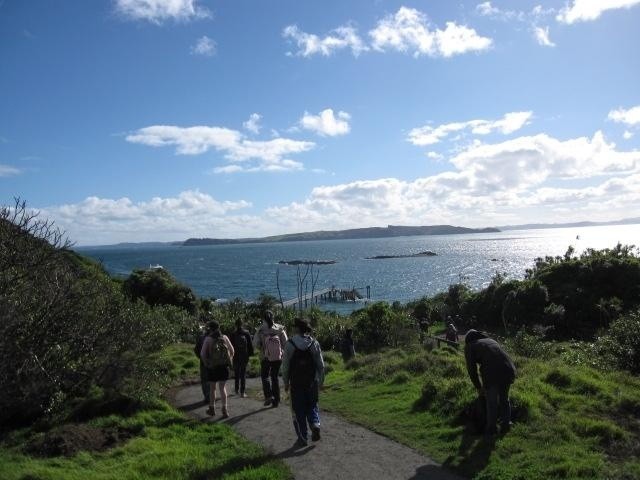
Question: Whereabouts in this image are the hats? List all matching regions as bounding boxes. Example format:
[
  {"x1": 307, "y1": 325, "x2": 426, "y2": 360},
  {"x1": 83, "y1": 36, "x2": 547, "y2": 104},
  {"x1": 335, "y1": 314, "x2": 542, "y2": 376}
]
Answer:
[{"x1": 206, "y1": 320, "x2": 220, "y2": 328}]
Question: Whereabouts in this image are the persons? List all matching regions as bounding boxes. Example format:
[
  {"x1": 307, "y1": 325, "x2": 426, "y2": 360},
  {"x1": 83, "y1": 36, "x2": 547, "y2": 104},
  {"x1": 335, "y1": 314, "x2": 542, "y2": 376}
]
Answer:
[
  {"x1": 281, "y1": 317, "x2": 326, "y2": 447},
  {"x1": 229, "y1": 318, "x2": 254, "y2": 398},
  {"x1": 418, "y1": 317, "x2": 429, "y2": 342},
  {"x1": 454, "y1": 314, "x2": 465, "y2": 329},
  {"x1": 341, "y1": 329, "x2": 357, "y2": 364},
  {"x1": 254, "y1": 309, "x2": 290, "y2": 409},
  {"x1": 446, "y1": 316, "x2": 459, "y2": 341},
  {"x1": 445, "y1": 324, "x2": 459, "y2": 354},
  {"x1": 463, "y1": 328, "x2": 518, "y2": 436},
  {"x1": 200, "y1": 320, "x2": 235, "y2": 418},
  {"x1": 193, "y1": 326, "x2": 211, "y2": 402}
]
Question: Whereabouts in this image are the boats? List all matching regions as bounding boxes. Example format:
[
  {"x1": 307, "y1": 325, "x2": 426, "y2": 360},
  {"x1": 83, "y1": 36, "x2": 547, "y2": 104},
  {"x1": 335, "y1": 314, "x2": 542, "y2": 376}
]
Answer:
[{"x1": 150, "y1": 264, "x2": 163, "y2": 270}]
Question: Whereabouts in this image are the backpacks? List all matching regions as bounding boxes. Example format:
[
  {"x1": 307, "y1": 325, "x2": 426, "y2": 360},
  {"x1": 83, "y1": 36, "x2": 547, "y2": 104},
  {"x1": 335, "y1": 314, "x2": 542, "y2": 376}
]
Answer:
[
  {"x1": 211, "y1": 334, "x2": 231, "y2": 367},
  {"x1": 288, "y1": 339, "x2": 317, "y2": 384},
  {"x1": 234, "y1": 330, "x2": 249, "y2": 354},
  {"x1": 259, "y1": 327, "x2": 283, "y2": 362}
]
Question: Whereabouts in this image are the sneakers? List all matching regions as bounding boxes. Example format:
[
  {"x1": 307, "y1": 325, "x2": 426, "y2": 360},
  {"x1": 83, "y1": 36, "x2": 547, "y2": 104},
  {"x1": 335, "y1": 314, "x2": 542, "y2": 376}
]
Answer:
[
  {"x1": 273, "y1": 400, "x2": 279, "y2": 407},
  {"x1": 241, "y1": 393, "x2": 249, "y2": 398},
  {"x1": 264, "y1": 399, "x2": 272, "y2": 405},
  {"x1": 223, "y1": 410, "x2": 229, "y2": 417},
  {"x1": 311, "y1": 424, "x2": 321, "y2": 441},
  {"x1": 206, "y1": 408, "x2": 215, "y2": 416},
  {"x1": 295, "y1": 439, "x2": 308, "y2": 446}
]
